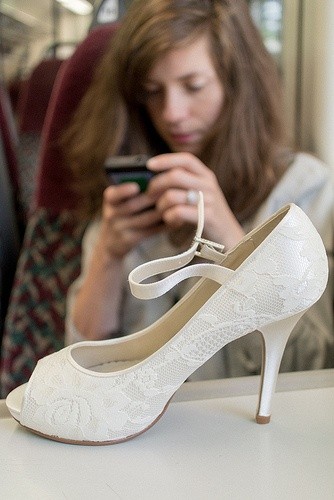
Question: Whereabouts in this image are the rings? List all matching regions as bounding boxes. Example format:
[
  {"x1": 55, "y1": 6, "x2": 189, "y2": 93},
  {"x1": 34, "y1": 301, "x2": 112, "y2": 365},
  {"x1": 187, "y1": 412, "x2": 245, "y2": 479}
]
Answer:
[{"x1": 186, "y1": 189, "x2": 197, "y2": 205}]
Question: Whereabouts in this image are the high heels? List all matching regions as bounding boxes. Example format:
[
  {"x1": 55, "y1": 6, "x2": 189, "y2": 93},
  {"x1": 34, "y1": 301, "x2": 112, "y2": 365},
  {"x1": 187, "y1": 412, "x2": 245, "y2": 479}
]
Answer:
[{"x1": 6, "y1": 190, "x2": 328, "y2": 446}]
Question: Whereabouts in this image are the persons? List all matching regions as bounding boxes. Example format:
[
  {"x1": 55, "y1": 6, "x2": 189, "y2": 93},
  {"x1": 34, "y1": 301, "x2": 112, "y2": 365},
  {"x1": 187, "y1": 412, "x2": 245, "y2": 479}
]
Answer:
[{"x1": 59, "y1": 0, "x2": 334, "y2": 382}]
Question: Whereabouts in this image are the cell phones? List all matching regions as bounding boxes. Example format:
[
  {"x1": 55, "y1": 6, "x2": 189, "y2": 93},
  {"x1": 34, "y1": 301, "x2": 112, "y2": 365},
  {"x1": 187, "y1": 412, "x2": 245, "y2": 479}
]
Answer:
[{"x1": 104, "y1": 155, "x2": 165, "y2": 227}]
